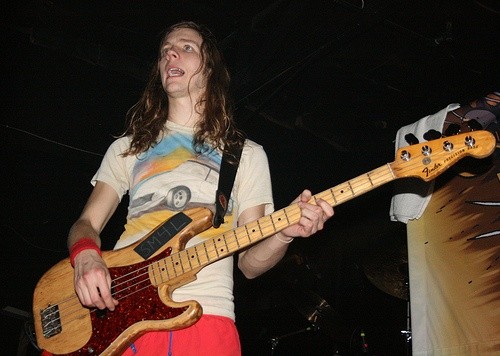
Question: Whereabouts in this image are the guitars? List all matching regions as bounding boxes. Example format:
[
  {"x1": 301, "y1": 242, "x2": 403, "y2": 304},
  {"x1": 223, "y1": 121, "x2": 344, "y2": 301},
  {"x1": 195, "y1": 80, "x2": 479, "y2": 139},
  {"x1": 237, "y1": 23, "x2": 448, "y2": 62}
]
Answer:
[{"x1": 34, "y1": 120, "x2": 496, "y2": 356}]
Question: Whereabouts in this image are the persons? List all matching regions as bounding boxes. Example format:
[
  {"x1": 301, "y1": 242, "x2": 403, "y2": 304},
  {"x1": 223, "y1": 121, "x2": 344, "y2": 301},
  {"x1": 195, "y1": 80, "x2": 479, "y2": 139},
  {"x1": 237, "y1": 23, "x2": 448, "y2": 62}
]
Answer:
[{"x1": 66, "y1": 20, "x2": 335, "y2": 356}]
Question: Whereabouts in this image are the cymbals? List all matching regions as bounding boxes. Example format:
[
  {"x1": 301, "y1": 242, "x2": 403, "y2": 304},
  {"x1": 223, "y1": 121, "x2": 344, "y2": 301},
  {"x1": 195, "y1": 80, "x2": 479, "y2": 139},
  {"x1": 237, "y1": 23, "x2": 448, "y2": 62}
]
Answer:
[
  {"x1": 362, "y1": 246, "x2": 408, "y2": 303},
  {"x1": 285, "y1": 279, "x2": 354, "y2": 342}
]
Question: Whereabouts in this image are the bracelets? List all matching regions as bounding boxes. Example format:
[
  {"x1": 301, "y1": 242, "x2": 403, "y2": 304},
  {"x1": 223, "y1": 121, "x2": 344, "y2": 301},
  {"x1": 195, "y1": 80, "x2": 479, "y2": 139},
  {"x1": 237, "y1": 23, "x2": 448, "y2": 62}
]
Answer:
[
  {"x1": 69, "y1": 237, "x2": 104, "y2": 269},
  {"x1": 275, "y1": 233, "x2": 294, "y2": 244}
]
